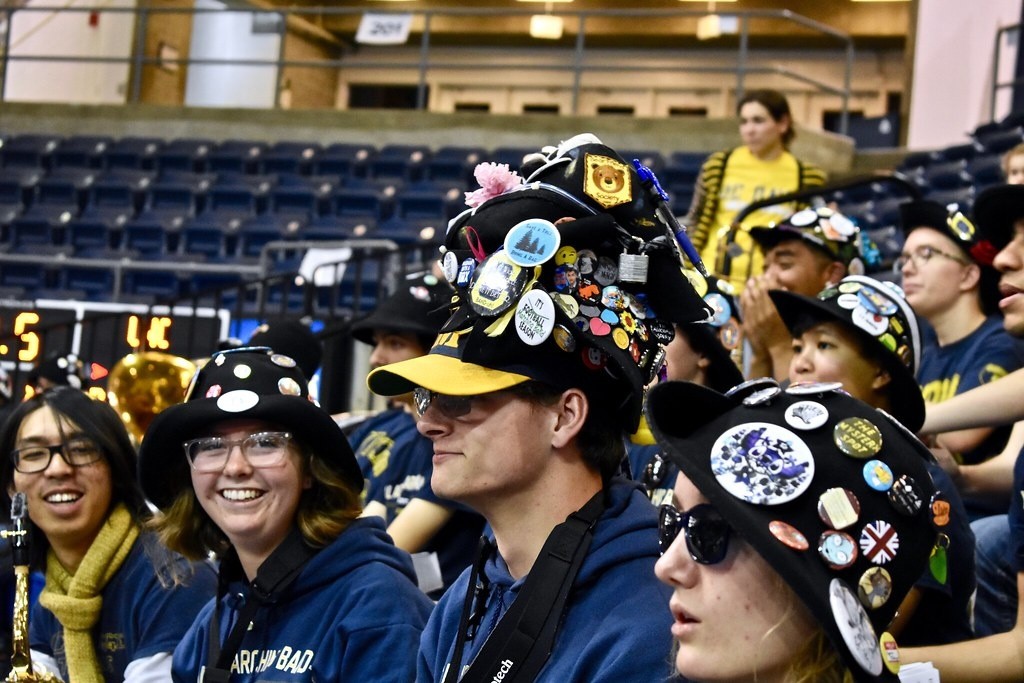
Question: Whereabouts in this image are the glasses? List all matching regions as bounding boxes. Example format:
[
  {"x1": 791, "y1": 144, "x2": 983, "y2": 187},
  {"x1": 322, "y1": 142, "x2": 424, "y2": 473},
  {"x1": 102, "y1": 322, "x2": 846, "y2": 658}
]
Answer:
[
  {"x1": 658, "y1": 503, "x2": 743, "y2": 565},
  {"x1": 413, "y1": 387, "x2": 565, "y2": 418},
  {"x1": 9, "y1": 438, "x2": 103, "y2": 473},
  {"x1": 892, "y1": 245, "x2": 968, "y2": 275},
  {"x1": 182, "y1": 431, "x2": 296, "y2": 473}
]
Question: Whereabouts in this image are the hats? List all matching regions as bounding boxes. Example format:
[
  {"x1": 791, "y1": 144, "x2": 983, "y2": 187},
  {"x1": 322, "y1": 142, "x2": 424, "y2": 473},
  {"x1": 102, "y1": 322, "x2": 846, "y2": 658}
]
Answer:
[
  {"x1": 212, "y1": 318, "x2": 322, "y2": 383},
  {"x1": 974, "y1": 184, "x2": 1024, "y2": 250},
  {"x1": 350, "y1": 274, "x2": 460, "y2": 350},
  {"x1": 677, "y1": 276, "x2": 745, "y2": 394},
  {"x1": 366, "y1": 134, "x2": 715, "y2": 434},
  {"x1": 898, "y1": 200, "x2": 1001, "y2": 317},
  {"x1": 136, "y1": 354, "x2": 364, "y2": 551},
  {"x1": 747, "y1": 206, "x2": 882, "y2": 277},
  {"x1": 767, "y1": 275, "x2": 925, "y2": 435},
  {"x1": 644, "y1": 377, "x2": 976, "y2": 683}
]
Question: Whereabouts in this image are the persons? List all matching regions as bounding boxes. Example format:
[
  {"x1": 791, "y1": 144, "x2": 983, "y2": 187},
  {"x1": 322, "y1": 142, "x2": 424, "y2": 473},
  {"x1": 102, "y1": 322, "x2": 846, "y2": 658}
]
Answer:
[
  {"x1": 684, "y1": 88, "x2": 831, "y2": 289},
  {"x1": 0, "y1": 119, "x2": 1024, "y2": 683}
]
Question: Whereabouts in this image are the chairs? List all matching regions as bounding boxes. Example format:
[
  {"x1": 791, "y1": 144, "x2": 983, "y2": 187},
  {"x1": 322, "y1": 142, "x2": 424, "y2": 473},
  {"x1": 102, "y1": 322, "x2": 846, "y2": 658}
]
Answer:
[
  {"x1": 833, "y1": 126, "x2": 1024, "y2": 286},
  {"x1": 0, "y1": 134, "x2": 711, "y2": 318}
]
